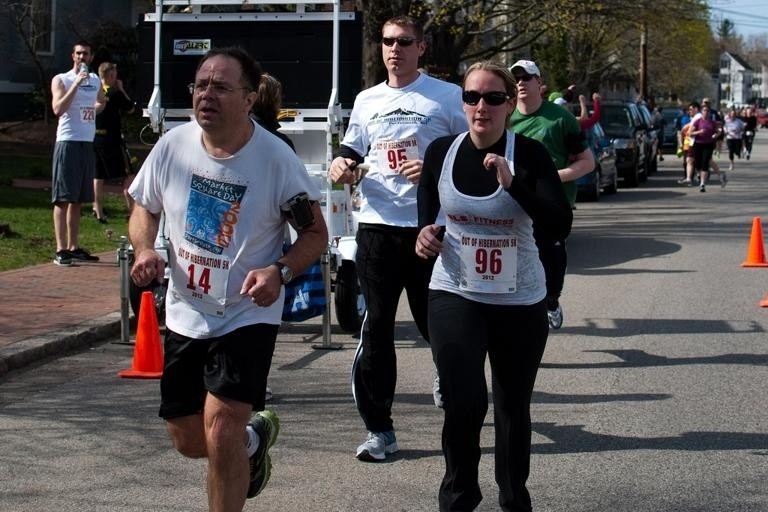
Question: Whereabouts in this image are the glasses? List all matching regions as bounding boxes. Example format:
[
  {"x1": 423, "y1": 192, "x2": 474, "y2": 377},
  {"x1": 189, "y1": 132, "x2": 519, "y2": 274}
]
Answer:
[
  {"x1": 514, "y1": 73, "x2": 535, "y2": 82},
  {"x1": 462, "y1": 87, "x2": 513, "y2": 106},
  {"x1": 382, "y1": 36, "x2": 422, "y2": 47},
  {"x1": 188, "y1": 82, "x2": 251, "y2": 99}
]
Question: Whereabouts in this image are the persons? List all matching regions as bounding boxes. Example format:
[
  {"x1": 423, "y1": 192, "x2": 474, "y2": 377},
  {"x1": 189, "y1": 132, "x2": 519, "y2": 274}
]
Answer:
[
  {"x1": 251, "y1": 71, "x2": 297, "y2": 405},
  {"x1": 686, "y1": 104, "x2": 722, "y2": 192},
  {"x1": 411, "y1": 60, "x2": 574, "y2": 512},
  {"x1": 50, "y1": 39, "x2": 106, "y2": 268},
  {"x1": 325, "y1": 13, "x2": 471, "y2": 463},
  {"x1": 128, "y1": 46, "x2": 330, "y2": 512},
  {"x1": 539, "y1": 81, "x2": 603, "y2": 139},
  {"x1": 91, "y1": 61, "x2": 135, "y2": 223},
  {"x1": 635, "y1": 92, "x2": 768, "y2": 188},
  {"x1": 508, "y1": 58, "x2": 596, "y2": 329}
]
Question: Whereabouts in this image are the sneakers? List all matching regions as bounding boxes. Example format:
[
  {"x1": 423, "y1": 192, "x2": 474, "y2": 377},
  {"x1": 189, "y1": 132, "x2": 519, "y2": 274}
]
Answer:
[
  {"x1": 433, "y1": 368, "x2": 444, "y2": 408},
  {"x1": 69, "y1": 249, "x2": 99, "y2": 263},
  {"x1": 546, "y1": 305, "x2": 563, "y2": 330},
  {"x1": 54, "y1": 250, "x2": 73, "y2": 267},
  {"x1": 700, "y1": 183, "x2": 706, "y2": 192},
  {"x1": 355, "y1": 430, "x2": 399, "y2": 461},
  {"x1": 718, "y1": 171, "x2": 727, "y2": 188},
  {"x1": 246, "y1": 409, "x2": 280, "y2": 499},
  {"x1": 678, "y1": 179, "x2": 692, "y2": 187}
]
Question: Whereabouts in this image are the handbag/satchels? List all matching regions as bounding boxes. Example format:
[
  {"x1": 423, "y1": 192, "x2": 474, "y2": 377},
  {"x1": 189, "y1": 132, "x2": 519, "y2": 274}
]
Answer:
[{"x1": 282, "y1": 245, "x2": 326, "y2": 321}]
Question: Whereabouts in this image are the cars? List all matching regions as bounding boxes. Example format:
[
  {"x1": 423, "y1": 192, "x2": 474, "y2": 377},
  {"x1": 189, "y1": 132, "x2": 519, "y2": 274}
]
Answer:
[
  {"x1": 568, "y1": 112, "x2": 618, "y2": 202},
  {"x1": 650, "y1": 105, "x2": 688, "y2": 153},
  {"x1": 594, "y1": 98, "x2": 660, "y2": 184}
]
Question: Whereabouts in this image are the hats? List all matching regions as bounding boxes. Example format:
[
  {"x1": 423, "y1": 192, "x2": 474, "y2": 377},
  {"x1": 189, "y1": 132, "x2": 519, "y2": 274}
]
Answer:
[{"x1": 509, "y1": 60, "x2": 540, "y2": 78}]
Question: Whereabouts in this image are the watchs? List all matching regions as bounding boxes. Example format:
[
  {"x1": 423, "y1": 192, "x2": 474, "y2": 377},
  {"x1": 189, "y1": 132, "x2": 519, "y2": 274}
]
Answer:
[{"x1": 272, "y1": 260, "x2": 295, "y2": 284}]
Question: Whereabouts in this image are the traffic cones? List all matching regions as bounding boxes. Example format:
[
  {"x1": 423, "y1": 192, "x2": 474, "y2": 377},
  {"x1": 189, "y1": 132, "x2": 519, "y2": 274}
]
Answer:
[
  {"x1": 739, "y1": 216, "x2": 768, "y2": 267},
  {"x1": 117, "y1": 290, "x2": 165, "y2": 381},
  {"x1": 759, "y1": 290, "x2": 767, "y2": 309}
]
